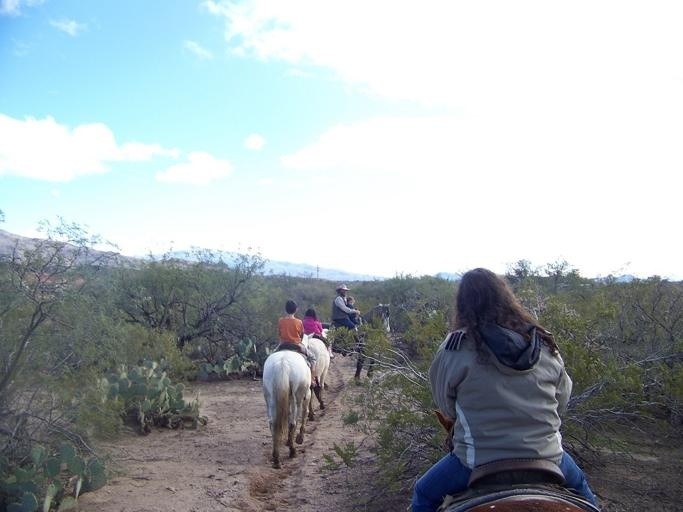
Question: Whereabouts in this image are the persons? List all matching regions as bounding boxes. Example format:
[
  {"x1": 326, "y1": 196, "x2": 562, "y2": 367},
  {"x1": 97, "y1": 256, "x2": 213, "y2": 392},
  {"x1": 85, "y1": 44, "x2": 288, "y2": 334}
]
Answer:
[
  {"x1": 270, "y1": 299, "x2": 318, "y2": 390},
  {"x1": 300, "y1": 306, "x2": 327, "y2": 350},
  {"x1": 330, "y1": 282, "x2": 363, "y2": 343},
  {"x1": 345, "y1": 293, "x2": 363, "y2": 326},
  {"x1": 399, "y1": 265, "x2": 602, "y2": 510}
]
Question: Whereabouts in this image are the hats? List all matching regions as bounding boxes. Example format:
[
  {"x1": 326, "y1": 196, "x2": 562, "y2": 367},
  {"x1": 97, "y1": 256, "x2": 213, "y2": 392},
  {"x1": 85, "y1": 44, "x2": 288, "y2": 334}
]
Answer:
[{"x1": 335, "y1": 283, "x2": 350, "y2": 293}]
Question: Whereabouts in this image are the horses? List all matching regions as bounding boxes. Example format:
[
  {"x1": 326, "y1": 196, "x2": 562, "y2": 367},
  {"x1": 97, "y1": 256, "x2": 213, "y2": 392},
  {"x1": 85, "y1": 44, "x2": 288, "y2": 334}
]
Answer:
[
  {"x1": 321, "y1": 302, "x2": 391, "y2": 385},
  {"x1": 262, "y1": 322, "x2": 334, "y2": 470},
  {"x1": 432, "y1": 409, "x2": 599, "y2": 512}
]
